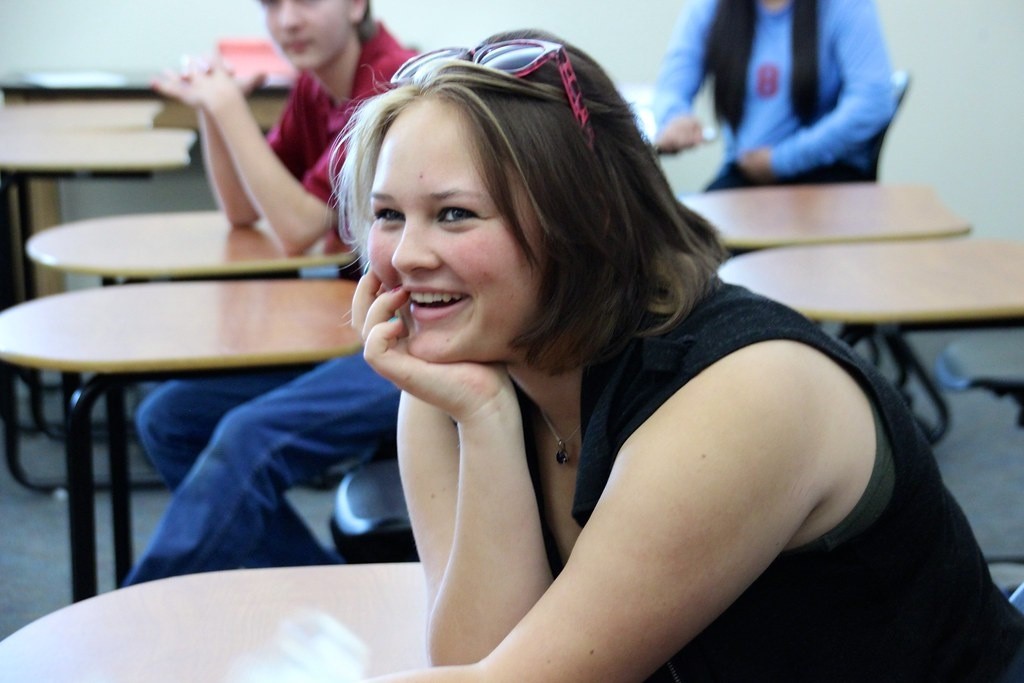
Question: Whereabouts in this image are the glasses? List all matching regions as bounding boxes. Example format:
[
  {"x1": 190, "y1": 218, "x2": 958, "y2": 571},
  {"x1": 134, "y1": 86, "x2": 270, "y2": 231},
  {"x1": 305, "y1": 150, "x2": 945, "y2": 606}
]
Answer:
[{"x1": 391, "y1": 39, "x2": 595, "y2": 155}]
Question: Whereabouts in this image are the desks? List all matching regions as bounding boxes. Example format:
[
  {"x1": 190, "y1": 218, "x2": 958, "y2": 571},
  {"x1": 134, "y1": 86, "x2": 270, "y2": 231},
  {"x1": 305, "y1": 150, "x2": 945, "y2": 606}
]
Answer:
[{"x1": 0, "y1": 93, "x2": 1023, "y2": 683}]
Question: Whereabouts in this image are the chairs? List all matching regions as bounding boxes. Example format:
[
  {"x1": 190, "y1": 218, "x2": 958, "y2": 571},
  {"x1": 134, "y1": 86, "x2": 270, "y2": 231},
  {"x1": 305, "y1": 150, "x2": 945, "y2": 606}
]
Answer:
[{"x1": 837, "y1": 75, "x2": 948, "y2": 444}]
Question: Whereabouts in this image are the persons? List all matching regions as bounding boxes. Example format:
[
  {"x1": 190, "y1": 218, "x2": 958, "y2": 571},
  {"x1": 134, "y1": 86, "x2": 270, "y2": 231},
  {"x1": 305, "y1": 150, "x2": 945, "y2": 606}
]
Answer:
[
  {"x1": 120, "y1": 1, "x2": 426, "y2": 584},
  {"x1": 331, "y1": 29, "x2": 1024, "y2": 683},
  {"x1": 648, "y1": 1, "x2": 901, "y2": 191}
]
